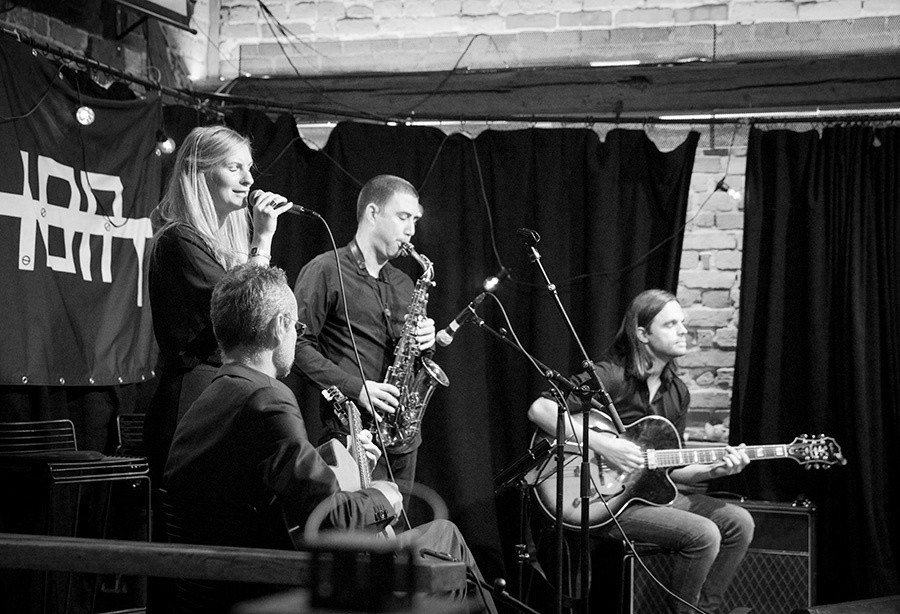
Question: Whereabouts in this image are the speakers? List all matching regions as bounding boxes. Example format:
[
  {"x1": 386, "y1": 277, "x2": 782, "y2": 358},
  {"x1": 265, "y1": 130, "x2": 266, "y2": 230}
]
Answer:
[{"x1": 592, "y1": 501, "x2": 817, "y2": 614}]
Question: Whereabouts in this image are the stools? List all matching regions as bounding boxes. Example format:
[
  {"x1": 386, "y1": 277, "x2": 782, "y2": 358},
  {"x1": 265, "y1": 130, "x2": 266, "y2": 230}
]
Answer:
[{"x1": 606, "y1": 533, "x2": 661, "y2": 614}]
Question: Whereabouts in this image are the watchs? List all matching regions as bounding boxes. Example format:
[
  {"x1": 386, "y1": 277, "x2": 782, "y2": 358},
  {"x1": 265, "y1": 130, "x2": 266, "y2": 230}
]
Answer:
[{"x1": 247, "y1": 246, "x2": 272, "y2": 261}]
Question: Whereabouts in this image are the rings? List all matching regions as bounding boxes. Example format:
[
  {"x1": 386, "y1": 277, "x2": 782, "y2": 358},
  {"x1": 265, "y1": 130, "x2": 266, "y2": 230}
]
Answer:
[{"x1": 270, "y1": 200, "x2": 277, "y2": 209}]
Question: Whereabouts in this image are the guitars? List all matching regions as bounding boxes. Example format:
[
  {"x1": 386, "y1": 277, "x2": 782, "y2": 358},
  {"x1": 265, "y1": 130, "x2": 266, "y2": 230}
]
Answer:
[
  {"x1": 321, "y1": 383, "x2": 397, "y2": 541},
  {"x1": 528, "y1": 407, "x2": 848, "y2": 531}
]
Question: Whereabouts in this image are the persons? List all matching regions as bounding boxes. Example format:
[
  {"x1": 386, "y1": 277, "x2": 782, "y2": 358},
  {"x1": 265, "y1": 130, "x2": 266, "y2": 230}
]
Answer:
[
  {"x1": 527, "y1": 289, "x2": 755, "y2": 614},
  {"x1": 147, "y1": 125, "x2": 293, "y2": 497},
  {"x1": 157, "y1": 264, "x2": 499, "y2": 614},
  {"x1": 294, "y1": 175, "x2": 436, "y2": 535}
]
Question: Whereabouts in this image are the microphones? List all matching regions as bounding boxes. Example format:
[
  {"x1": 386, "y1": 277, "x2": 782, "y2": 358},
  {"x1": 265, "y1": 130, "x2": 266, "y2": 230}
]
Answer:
[
  {"x1": 436, "y1": 293, "x2": 486, "y2": 348},
  {"x1": 249, "y1": 189, "x2": 320, "y2": 219},
  {"x1": 482, "y1": 271, "x2": 510, "y2": 293}
]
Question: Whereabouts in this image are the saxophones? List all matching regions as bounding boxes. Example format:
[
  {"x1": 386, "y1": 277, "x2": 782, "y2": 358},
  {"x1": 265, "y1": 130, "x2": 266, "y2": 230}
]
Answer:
[{"x1": 370, "y1": 241, "x2": 450, "y2": 455}]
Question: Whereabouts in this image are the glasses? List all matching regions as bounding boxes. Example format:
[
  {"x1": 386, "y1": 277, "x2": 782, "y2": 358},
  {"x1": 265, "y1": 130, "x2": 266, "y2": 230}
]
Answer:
[{"x1": 267, "y1": 314, "x2": 307, "y2": 339}]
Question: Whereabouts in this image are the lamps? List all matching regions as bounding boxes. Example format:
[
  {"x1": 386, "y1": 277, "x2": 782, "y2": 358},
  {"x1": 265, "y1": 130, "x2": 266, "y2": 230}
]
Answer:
[
  {"x1": 157, "y1": 128, "x2": 176, "y2": 155},
  {"x1": 75, "y1": 106, "x2": 95, "y2": 126}
]
